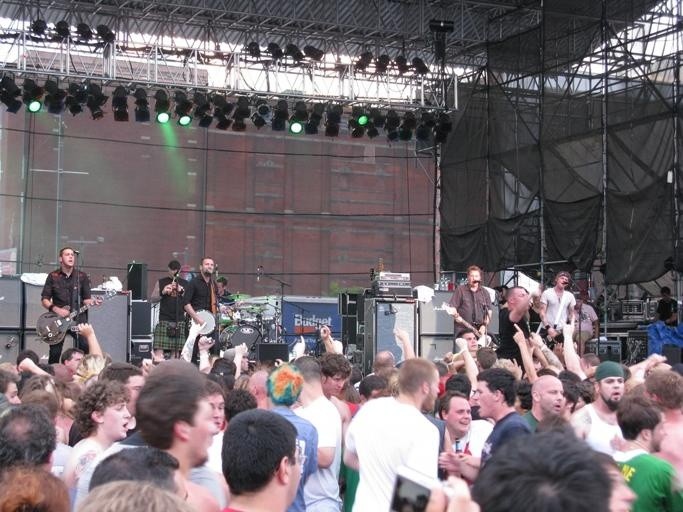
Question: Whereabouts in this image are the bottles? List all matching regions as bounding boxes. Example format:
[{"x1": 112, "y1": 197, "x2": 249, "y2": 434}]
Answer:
[
  {"x1": 102, "y1": 274, "x2": 106, "y2": 290},
  {"x1": 87, "y1": 272, "x2": 91, "y2": 289},
  {"x1": 439, "y1": 278, "x2": 449, "y2": 291},
  {"x1": 455, "y1": 438, "x2": 463, "y2": 454}
]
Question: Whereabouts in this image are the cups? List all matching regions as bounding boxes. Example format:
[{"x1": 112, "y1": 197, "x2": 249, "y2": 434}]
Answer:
[{"x1": 434, "y1": 284, "x2": 439, "y2": 290}]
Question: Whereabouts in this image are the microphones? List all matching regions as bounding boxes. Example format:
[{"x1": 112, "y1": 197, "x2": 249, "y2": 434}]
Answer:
[
  {"x1": 257, "y1": 265, "x2": 260, "y2": 281},
  {"x1": 474, "y1": 280, "x2": 482, "y2": 283},
  {"x1": 565, "y1": 282, "x2": 569, "y2": 284}
]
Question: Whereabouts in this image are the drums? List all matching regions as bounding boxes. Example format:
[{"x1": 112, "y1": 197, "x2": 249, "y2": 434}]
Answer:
[
  {"x1": 244, "y1": 320, "x2": 269, "y2": 343},
  {"x1": 220, "y1": 320, "x2": 265, "y2": 361}
]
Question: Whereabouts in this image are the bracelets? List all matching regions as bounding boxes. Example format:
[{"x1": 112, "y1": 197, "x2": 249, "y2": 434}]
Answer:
[
  {"x1": 49, "y1": 305, "x2": 55, "y2": 312},
  {"x1": 160, "y1": 290, "x2": 167, "y2": 297},
  {"x1": 178, "y1": 289, "x2": 183, "y2": 294}
]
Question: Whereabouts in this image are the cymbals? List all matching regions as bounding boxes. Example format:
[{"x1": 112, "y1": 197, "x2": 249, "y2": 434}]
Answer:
[
  {"x1": 239, "y1": 306, "x2": 270, "y2": 314},
  {"x1": 227, "y1": 293, "x2": 251, "y2": 300}
]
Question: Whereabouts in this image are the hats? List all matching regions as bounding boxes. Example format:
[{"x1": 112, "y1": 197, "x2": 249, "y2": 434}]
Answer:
[
  {"x1": 169, "y1": 261, "x2": 181, "y2": 270},
  {"x1": 266, "y1": 363, "x2": 305, "y2": 405}
]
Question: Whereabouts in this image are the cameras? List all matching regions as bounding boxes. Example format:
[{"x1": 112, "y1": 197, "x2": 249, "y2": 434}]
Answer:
[
  {"x1": 386, "y1": 465, "x2": 453, "y2": 512},
  {"x1": 71, "y1": 326, "x2": 79, "y2": 332}
]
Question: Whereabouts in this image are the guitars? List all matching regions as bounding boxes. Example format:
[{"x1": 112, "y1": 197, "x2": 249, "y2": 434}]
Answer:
[
  {"x1": 539, "y1": 328, "x2": 564, "y2": 347},
  {"x1": 37, "y1": 289, "x2": 117, "y2": 346},
  {"x1": 443, "y1": 303, "x2": 500, "y2": 354}
]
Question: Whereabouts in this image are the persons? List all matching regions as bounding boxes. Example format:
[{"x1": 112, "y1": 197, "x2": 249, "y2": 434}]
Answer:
[
  {"x1": 216, "y1": 277, "x2": 235, "y2": 307},
  {"x1": 150, "y1": 260, "x2": 190, "y2": 360},
  {"x1": 41, "y1": 247, "x2": 102, "y2": 366},
  {"x1": 654, "y1": 286, "x2": 678, "y2": 326},
  {"x1": 539, "y1": 270, "x2": 578, "y2": 352},
  {"x1": 1, "y1": 322, "x2": 683, "y2": 512},
  {"x1": 498, "y1": 286, "x2": 541, "y2": 373},
  {"x1": 450, "y1": 265, "x2": 492, "y2": 354},
  {"x1": 575, "y1": 296, "x2": 600, "y2": 358},
  {"x1": 183, "y1": 257, "x2": 220, "y2": 365}
]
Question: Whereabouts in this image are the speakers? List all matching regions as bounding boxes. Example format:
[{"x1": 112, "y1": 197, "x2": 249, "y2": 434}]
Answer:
[
  {"x1": 356, "y1": 295, "x2": 364, "y2": 350},
  {"x1": 127, "y1": 263, "x2": 148, "y2": 299},
  {"x1": 257, "y1": 343, "x2": 289, "y2": 363}
]
